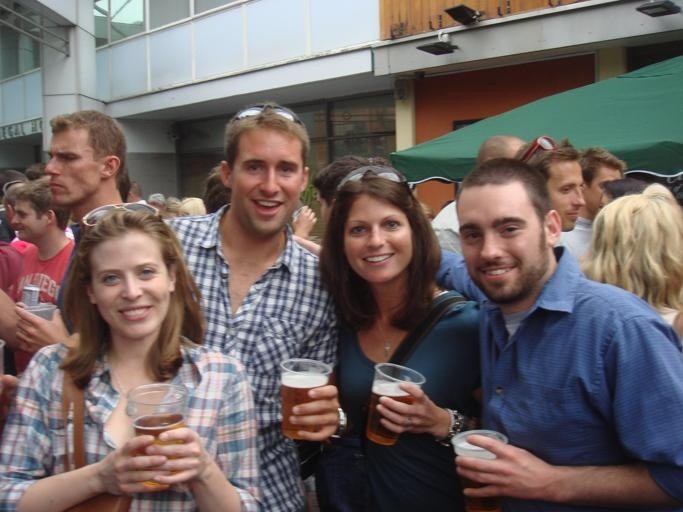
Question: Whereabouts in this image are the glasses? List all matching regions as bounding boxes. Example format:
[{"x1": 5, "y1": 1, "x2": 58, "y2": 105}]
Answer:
[
  {"x1": 336, "y1": 165, "x2": 408, "y2": 191},
  {"x1": 229, "y1": 106, "x2": 300, "y2": 122},
  {"x1": 2, "y1": 179, "x2": 22, "y2": 197},
  {"x1": 521, "y1": 135, "x2": 556, "y2": 163},
  {"x1": 81, "y1": 202, "x2": 159, "y2": 227}
]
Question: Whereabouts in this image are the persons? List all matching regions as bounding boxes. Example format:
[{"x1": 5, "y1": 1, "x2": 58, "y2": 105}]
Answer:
[
  {"x1": 127, "y1": 179, "x2": 148, "y2": 204},
  {"x1": 429, "y1": 135, "x2": 528, "y2": 254},
  {"x1": 433, "y1": 157, "x2": 683, "y2": 512},
  {"x1": 514, "y1": 142, "x2": 586, "y2": 233},
  {"x1": 2, "y1": 182, "x2": 76, "y2": 253},
  {"x1": 290, "y1": 155, "x2": 392, "y2": 257},
  {"x1": 0, "y1": 205, "x2": 260, "y2": 512},
  {"x1": 580, "y1": 193, "x2": 683, "y2": 343},
  {"x1": 562, "y1": 147, "x2": 625, "y2": 266},
  {"x1": 314, "y1": 164, "x2": 483, "y2": 512},
  {"x1": 7, "y1": 178, "x2": 74, "y2": 375},
  {"x1": 13, "y1": 109, "x2": 127, "y2": 356},
  {"x1": 160, "y1": 102, "x2": 342, "y2": 512},
  {"x1": 205, "y1": 165, "x2": 318, "y2": 240}
]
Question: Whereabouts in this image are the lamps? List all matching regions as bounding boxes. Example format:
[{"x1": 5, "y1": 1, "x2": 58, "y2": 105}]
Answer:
[
  {"x1": 443, "y1": 4, "x2": 480, "y2": 25},
  {"x1": 637, "y1": 1, "x2": 682, "y2": 17},
  {"x1": 415, "y1": 36, "x2": 459, "y2": 56}
]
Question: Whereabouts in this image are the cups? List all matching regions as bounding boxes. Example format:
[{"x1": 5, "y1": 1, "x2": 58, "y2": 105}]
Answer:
[
  {"x1": 280, "y1": 359, "x2": 332, "y2": 440},
  {"x1": 365, "y1": 364, "x2": 425, "y2": 445},
  {"x1": 455, "y1": 430, "x2": 508, "y2": 511},
  {"x1": 128, "y1": 383, "x2": 190, "y2": 487},
  {"x1": 24, "y1": 305, "x2": 57, "y2": 322}
]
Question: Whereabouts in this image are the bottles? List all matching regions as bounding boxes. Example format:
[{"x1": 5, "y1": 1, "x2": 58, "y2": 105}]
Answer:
[{"x1": 21, "y1": 284, "x2": 40, "y2": 306}]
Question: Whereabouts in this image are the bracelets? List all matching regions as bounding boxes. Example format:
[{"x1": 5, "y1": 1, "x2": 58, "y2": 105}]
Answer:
[
  {"x1": 331, "y1": 408, "x2": 348, "y2": 439},
  {"x1": 434, "y1": 408, "x2": 466, "y2": 447}
]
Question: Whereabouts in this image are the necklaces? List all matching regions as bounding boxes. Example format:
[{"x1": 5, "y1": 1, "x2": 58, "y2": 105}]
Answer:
[
  {"x1": 111, "y1": 364, "x2": 140, "y2": 418},
  {"x1": 376, "y1": 320, "x2": 399, "y2": 356}
]
